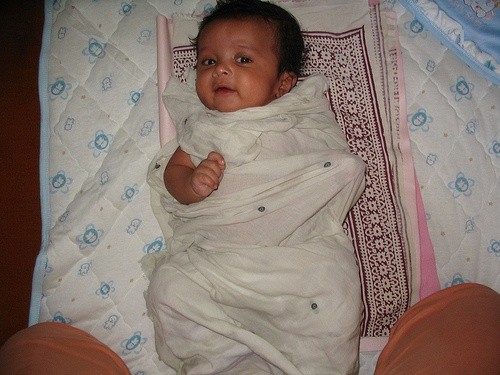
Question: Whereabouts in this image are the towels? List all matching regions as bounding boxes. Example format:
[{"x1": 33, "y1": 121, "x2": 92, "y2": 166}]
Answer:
[{"x1": 170, "y1": 5, "x2": 415, "y2": 338}]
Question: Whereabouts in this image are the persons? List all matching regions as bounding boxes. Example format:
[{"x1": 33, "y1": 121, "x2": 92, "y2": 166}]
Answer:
[{"x1": 143, "y1": 0, "x2": 368, "y2": 374}]
